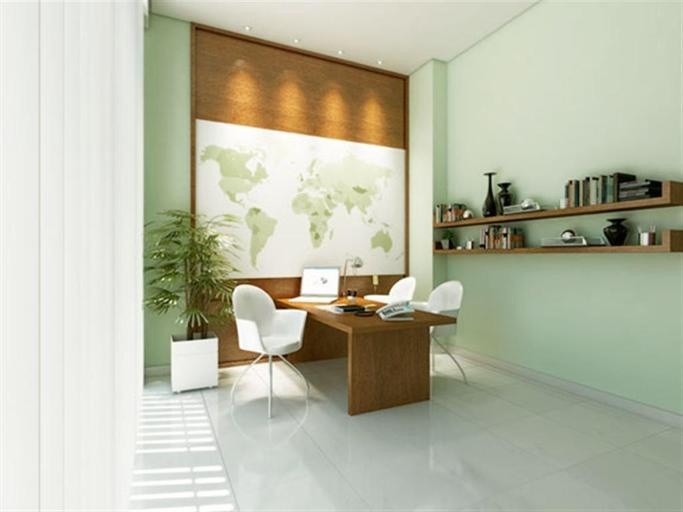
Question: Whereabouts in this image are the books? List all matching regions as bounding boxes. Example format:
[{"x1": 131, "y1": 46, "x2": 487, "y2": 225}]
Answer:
[
  {"x1": 565, "y1": 173, "x2": 661, "y2": 208},
  {"x1": 436, "y1": 203, "x2": 467, "y2": 223},
  {"x1": 480, "y1": 225, "x2": 523, "y2": 249}
]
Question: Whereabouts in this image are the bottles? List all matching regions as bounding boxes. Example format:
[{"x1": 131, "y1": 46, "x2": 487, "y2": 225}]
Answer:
[
  {"x1": 495, "y1": 182, "x2": 517, "y2": 215},
  {"x1": 602, "y1": 217, "x2": 630, "y2": 245},
  {"x1": 481, "y1": 172, "x2": 498, "y2": 218}
]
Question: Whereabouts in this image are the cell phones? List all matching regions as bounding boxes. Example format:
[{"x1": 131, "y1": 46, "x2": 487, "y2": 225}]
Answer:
[{"x1": 355, "y1": 311, "x2": 374, "y2": 317}]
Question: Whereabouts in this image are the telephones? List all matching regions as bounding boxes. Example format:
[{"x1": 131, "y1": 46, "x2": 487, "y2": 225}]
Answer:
[{"x1": 376, "y1": 300, "x2": 415, "y2": 321}]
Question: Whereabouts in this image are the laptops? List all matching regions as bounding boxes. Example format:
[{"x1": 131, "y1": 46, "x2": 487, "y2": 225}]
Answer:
[{"x1": 289, "y1": 267, "x2": 339, "y2": 304}]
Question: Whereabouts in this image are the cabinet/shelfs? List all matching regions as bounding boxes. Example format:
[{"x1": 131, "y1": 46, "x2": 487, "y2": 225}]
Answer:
[{"x1": 432, "y1": 180, "x2": 683, "y2": 255}]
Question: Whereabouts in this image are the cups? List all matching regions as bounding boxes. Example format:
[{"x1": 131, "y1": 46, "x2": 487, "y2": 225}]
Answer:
[{"x1": 637, "y1": 232, "x2": 656, "y2": 246}]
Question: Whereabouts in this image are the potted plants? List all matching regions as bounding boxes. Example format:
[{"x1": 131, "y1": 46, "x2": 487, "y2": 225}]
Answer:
[{"x1": 143, "y1": 207, "x2": 251, "y2": 397}]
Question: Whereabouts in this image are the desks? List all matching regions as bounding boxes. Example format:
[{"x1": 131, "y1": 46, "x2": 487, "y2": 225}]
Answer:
[{"x1": 274, "y1": 295, "x2": 456, "y2": 417}]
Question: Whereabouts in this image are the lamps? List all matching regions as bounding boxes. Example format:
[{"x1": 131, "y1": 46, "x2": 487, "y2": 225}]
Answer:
[{"x1": 341, "y1": 257, "x2": 363, "y2": 296}]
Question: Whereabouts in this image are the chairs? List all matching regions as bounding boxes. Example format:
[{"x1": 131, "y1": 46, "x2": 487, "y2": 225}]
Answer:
[
  {"x1": 363, "y1": 274, "x2": 418, "y2": 304},
  {"x1": 229, "y1": 282, "x2": 309, "y2": 421},
  {"x1": 407, "y1": 279, "x2": 468, "y2": 384}
]
